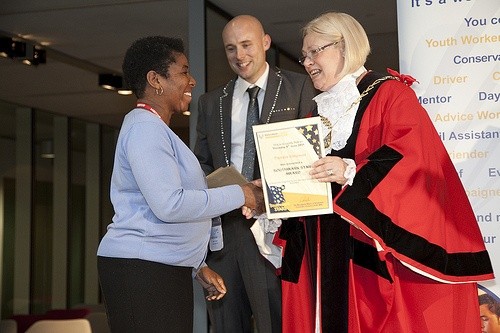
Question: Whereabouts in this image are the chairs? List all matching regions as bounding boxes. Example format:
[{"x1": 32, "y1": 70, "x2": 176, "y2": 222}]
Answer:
[{"x1": 0, "y1": 304, "x2": 110, "y2": 333}]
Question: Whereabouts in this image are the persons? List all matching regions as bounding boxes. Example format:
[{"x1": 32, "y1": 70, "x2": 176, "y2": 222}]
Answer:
[
  {"x1": 96, "y1": 36, "x2": 267, "y2": 333},
  {"x1": 242, "y1": 11, "x2": 494, "y2": 333},
  {"x1": 478, "y1": 294, "x2": 500, "y2": 333},
  {"x1": 194, "y1": 14, "x2": 324, "y2": 333}
]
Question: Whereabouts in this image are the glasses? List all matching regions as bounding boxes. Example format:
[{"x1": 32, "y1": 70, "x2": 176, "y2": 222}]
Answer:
[{"x1": 298, "y1": 37, "x2": 343, "y2": 65}]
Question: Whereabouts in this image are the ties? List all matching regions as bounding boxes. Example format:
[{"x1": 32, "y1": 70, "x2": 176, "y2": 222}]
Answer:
[{"x1": 241, "y1": 86, "x2": 260, "y2": 182}]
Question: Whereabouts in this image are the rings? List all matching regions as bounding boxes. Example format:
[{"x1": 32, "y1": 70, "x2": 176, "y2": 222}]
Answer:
[{"x1": 327, "y1": 170, "x2": 335, "y2": 175}]
